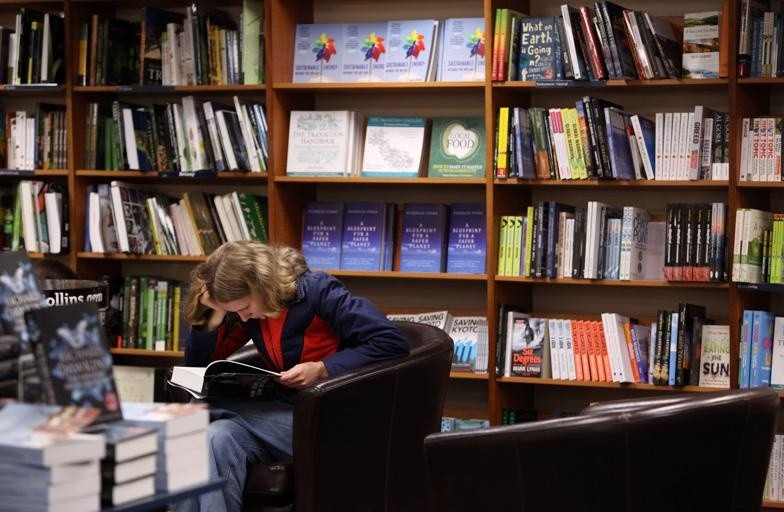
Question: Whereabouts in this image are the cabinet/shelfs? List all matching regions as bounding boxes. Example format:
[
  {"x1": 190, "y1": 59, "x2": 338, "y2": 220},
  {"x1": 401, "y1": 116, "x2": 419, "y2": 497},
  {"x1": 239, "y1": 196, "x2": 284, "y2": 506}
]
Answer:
[
  {"x1": 0, "y1": 0, "x2": 273, "y2": 400},
  {"x1": 269, "y1": 1, "x2": 784, "y2": 511}
]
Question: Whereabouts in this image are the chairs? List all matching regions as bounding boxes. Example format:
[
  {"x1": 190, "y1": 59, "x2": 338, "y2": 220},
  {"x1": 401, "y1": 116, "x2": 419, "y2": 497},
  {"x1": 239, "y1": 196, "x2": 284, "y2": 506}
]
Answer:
[
  {"x1": 152, "y1": 319, "x2": 454, "y2": 511},
  {"x1": 421, "y1": 386, "x2": 781, "y2": 511}
]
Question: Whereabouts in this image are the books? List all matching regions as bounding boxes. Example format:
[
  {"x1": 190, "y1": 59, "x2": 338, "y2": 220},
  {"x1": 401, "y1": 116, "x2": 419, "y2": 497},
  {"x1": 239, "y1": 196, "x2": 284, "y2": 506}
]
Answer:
[
  {"x1": 75, "y1": 2, "x2": 269, "y2": 349},
  {"x1": 0, "y1": 266, "x2": 211, "y2": 510},
  {"x1": 287, "y1": 16, "x2": 490, "y2": 374},
  {"x1": 764, "y1": 435, "x2": 783, "y2": 501},
  {"x1": 492, "y1": 1, "x2": 784, "y2": 386},
  {"x1": 2, "y1": 10, "x2": 70, "y2": 256},
  {"x1": 167, "y1": 360, "x2": 290, "y2": 403},
  {"x1": 440, "y1": 406, "x2": 578, "y2": 433}
]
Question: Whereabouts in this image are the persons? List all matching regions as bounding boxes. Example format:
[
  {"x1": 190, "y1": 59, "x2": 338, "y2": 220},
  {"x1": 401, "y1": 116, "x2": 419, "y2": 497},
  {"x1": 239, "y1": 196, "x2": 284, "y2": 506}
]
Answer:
[{"x1": 185, "y1": 241, "x2": 411, "y2": 512}]
